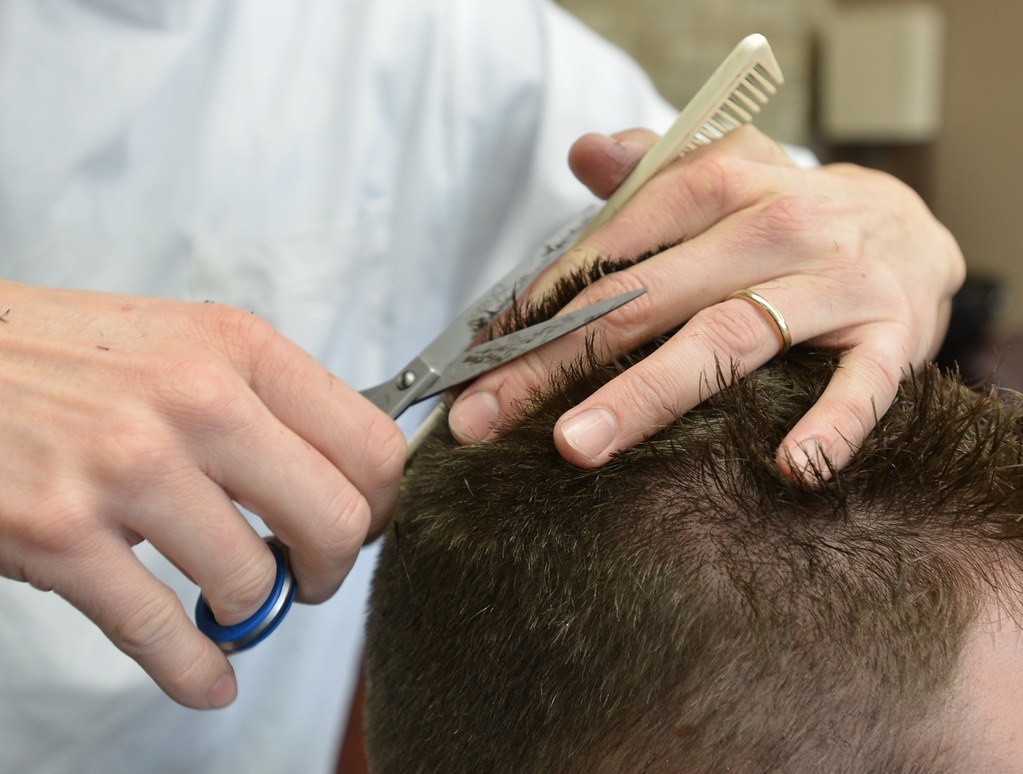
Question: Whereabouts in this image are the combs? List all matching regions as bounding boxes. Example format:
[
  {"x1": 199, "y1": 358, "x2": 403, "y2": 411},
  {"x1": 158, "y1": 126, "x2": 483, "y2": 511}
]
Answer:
[{"x1": 406, "y1": 33, "x2": 783, "y2": 463}]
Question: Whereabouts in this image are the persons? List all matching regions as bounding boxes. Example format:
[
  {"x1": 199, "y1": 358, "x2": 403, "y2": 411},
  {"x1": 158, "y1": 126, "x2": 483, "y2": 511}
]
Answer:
[
  {"x1": 0, "y1": 1, "x2": 967, "y2": 774},
  {"x1": 360, "y1": 344, "x2": 1022, "y2": 774}
]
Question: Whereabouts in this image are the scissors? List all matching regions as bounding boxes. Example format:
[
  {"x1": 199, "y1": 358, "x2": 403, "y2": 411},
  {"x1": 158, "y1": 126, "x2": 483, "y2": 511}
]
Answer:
[{"x1": 191, "y1": 201, "x2": 648, "y2": 664}]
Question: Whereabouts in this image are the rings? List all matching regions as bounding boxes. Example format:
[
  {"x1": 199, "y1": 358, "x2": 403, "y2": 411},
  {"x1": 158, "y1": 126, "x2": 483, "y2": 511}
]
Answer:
[{"x1": 725, "y1": 289, "x2": 792, "y2": 354}]
